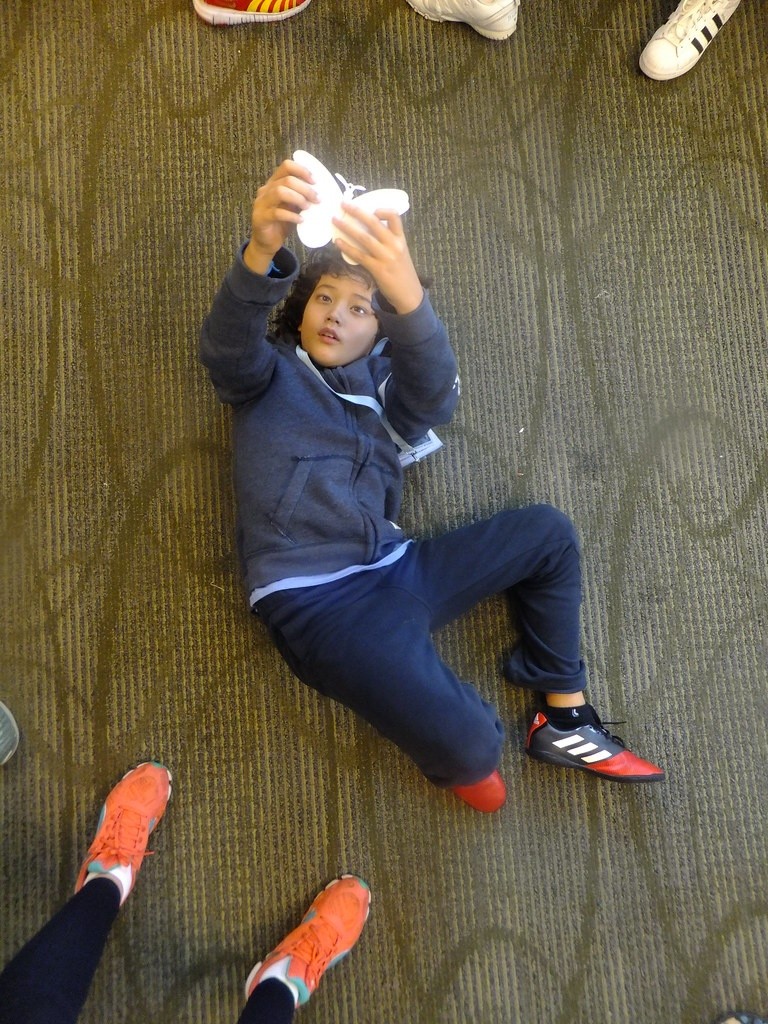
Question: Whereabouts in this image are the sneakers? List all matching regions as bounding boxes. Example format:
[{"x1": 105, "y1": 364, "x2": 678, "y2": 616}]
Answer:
[
  {"x1": 639, "y1": 0, "x2": 740, "y2": 81},
  {"x1": 193, "y1": 0, "x2": 311, "y2": 25},
  {"x1": 524, "y1": 709, "x2": 666, "y2": 781},
  {"x1": 407, "y1": 0, "x2": 522, "y2": 41},
  {"x1": 451, "y1": 770, "x2": 506, "y2": 812},
  {"x1": 72, "y1": 760, "x2": 172, "y2": 911},
  {"x1": 244, "y1": 876, "x2": 372, "y2": 1010}
]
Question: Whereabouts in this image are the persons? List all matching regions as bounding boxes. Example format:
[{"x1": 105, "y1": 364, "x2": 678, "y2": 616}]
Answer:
[
  {"x1": 405, "y1": 0, "x2": 742, "y2": 80},
  {"x1": 0, "y1": 760, "x2": 371, "y2": 1023},
  {"x1": 199, "y1": 158, "x2": 666, "y2": 812},
  {"x1": 193, "y1": 0, "x2": 309, "y2": 26}
]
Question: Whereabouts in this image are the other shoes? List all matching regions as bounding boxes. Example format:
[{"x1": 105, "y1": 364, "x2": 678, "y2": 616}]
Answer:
[{"x1": 0, "y1": 701, "x2": 20, "y2": 766}]
